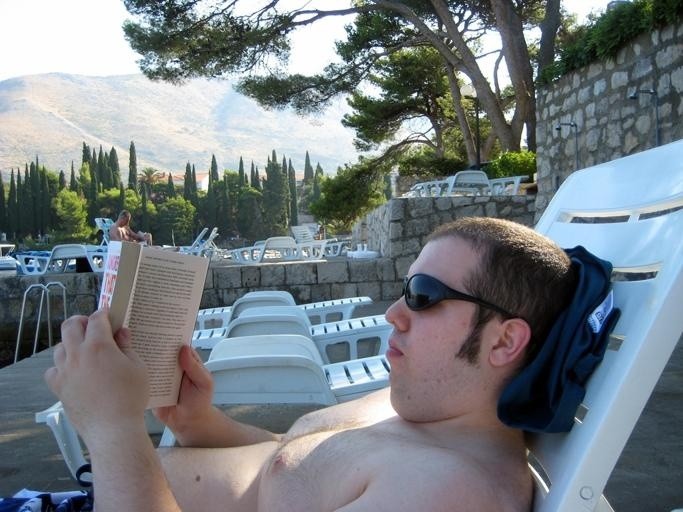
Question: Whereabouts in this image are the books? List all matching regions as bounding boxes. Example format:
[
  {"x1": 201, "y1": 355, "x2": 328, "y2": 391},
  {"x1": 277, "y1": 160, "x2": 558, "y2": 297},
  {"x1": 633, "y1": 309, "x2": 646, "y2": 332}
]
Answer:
[{"x1": 97, "y1": 239, "x2": 210, "y2": 410}]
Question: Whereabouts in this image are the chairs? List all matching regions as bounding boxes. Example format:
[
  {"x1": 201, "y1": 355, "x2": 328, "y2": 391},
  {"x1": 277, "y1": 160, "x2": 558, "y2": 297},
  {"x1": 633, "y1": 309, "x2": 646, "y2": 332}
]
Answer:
[{"x1": 0, "y1": 136, "x2": 682, "y2": 512}]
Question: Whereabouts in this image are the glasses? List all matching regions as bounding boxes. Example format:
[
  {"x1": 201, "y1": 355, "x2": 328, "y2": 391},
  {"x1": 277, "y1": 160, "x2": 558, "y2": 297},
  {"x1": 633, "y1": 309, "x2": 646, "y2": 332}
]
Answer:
[{"x1": 398, "y1": 273, "x2": 513, "y2": 320}]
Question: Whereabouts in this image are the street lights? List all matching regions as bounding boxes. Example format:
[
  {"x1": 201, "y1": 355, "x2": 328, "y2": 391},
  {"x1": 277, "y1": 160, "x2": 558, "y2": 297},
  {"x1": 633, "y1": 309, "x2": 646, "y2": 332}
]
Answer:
[{"x1": 459, "y1": 84, "x2": 497, "y2": 170}]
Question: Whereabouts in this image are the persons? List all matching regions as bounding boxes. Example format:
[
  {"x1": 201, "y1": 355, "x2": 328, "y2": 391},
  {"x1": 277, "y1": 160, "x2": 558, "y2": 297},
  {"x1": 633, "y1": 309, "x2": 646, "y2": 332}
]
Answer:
[
  {"x1": 0, "y1": 215, "x2": 578, "y2": 512},
  {"x1": 109, "y1": 209, "x2": 146, "y2": 242}
]
